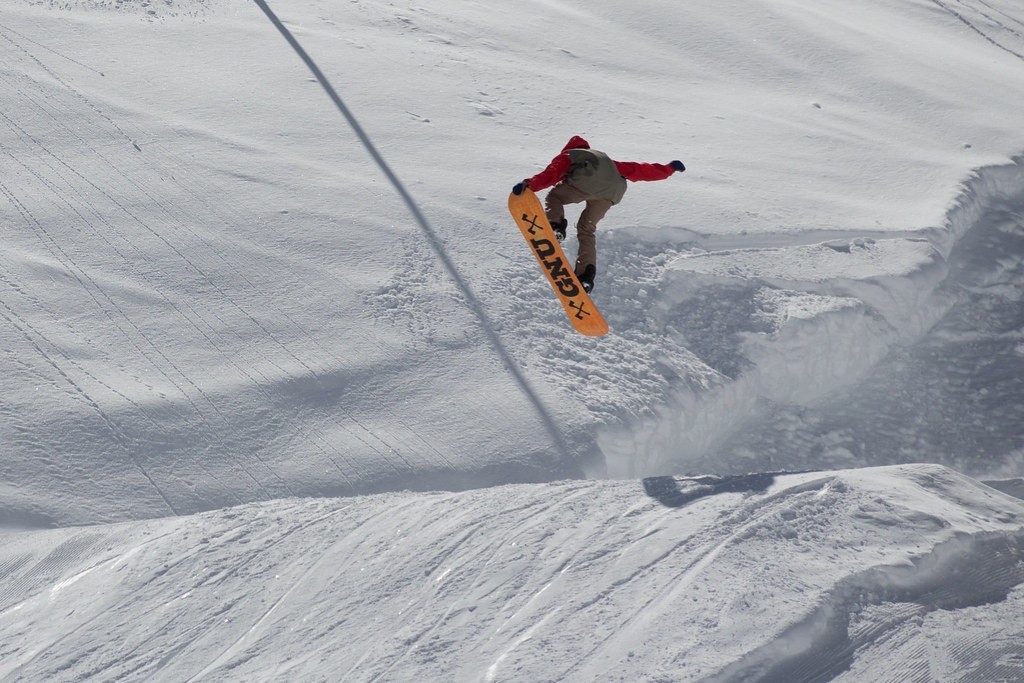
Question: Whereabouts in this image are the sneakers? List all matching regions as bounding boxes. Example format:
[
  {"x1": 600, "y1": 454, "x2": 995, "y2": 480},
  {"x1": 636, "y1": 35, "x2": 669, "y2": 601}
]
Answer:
[
  {"x1": 549, "y1": 218, "x2": 567, "y2": 241},
  {"x1": 579, "y1": 277, "x2": 594, "y2": 294}
]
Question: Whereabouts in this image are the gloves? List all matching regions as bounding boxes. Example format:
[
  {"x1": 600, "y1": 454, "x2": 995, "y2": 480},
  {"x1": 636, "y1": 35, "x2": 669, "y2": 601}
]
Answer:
[
  {"x1": 513, "y1": 182, "x2": 525, "y2": 195},
  {"x1": 670, "y1": 160, "x2": 685, "y2": 172}
]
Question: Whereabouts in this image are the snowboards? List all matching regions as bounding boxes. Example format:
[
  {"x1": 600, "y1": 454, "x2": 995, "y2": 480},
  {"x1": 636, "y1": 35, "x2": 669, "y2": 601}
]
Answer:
[{"x1": 507, "y1": 186, "x2": 609, "y2": 337}]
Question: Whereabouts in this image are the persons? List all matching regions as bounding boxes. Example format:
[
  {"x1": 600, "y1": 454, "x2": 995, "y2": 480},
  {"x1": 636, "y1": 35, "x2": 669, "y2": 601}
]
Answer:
[{"x1": 512, "y1": 136, "x2": 686, "y2": 295}]
